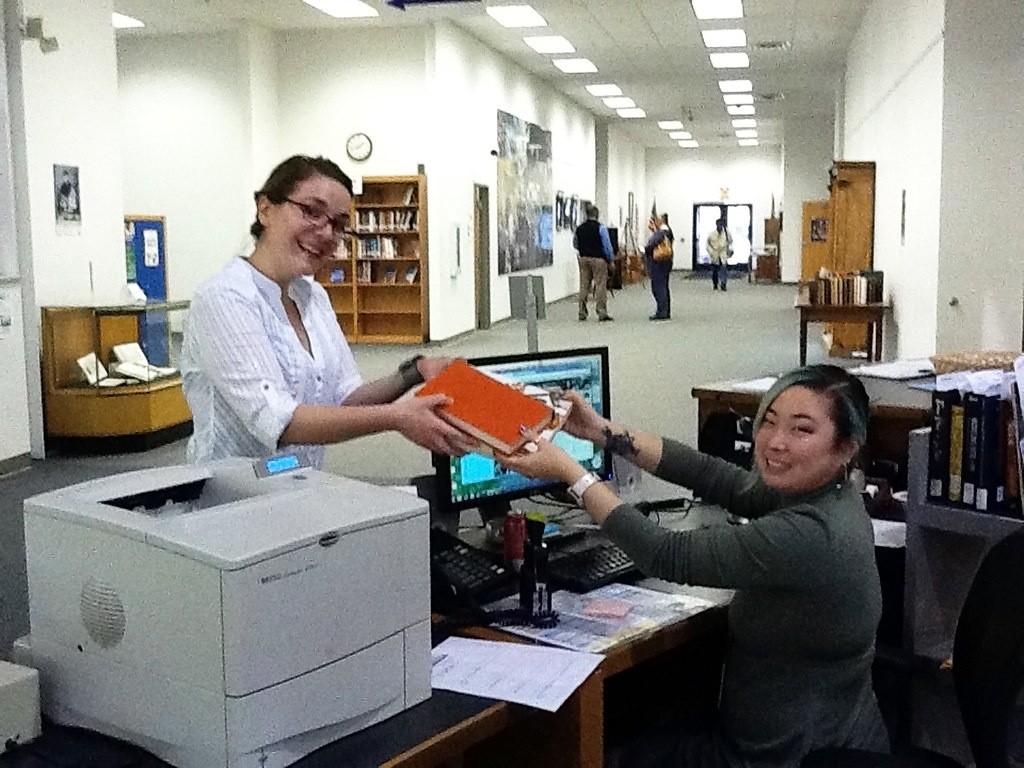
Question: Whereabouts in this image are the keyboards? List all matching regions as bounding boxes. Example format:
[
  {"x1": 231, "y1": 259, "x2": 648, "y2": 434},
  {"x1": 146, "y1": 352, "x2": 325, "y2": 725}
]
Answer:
[{"x1": 548, "y1": 539, "x2": 637, "y2": 593}]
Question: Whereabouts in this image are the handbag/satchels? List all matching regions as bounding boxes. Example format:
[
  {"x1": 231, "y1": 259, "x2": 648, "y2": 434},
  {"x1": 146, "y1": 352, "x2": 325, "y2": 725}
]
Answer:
[
  {"x1": 653, "y1": 236, "x2": 672, "y2": 261},
  {"x1": 727, "y1": 249, "x2": 734, "y2": 259}
]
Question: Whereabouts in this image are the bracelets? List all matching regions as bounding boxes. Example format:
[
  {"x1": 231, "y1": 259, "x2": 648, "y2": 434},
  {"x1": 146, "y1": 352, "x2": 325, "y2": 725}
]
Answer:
[{"x1": 399, "y1": 354, "x2": 426, "y2": 388}]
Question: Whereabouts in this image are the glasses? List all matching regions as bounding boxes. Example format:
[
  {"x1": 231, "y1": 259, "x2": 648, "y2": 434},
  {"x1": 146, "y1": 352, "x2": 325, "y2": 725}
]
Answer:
[{"x1": 285, "y1": 197, "x2": 358, "y2": 241}]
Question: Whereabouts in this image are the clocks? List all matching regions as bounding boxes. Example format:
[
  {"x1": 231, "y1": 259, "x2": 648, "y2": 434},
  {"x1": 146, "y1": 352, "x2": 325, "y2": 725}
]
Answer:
[{"x1": 346, "y1": 133, "x2": 372, "y2": 160}]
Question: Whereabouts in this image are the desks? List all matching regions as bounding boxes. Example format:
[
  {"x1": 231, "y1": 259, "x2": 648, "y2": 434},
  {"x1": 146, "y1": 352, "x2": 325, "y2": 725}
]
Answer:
[
  {"x1": 796, "y1": 304, "x2": 888, "y2": 367},
  {"x1": 692, "y1": 360, "x2": 933, "y2": 488},
  {"x1": 381, "y1": 603, "x2": 730, "y2": 768}
]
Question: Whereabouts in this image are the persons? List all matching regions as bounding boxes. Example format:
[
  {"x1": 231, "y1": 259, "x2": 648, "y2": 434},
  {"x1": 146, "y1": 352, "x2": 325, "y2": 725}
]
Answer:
[
  {"x1": 497, "y1": 363, "x2": 892, "y2": 768},
  {"x1": 645, "y1": 217, "x2": 672, "y2": 320},
  {"x1": 573, "y1": 207, "x2": 612, "y2": 321},
  {"x1": 707, "y1": 219, "x2": 733, "y2": 292},
  {"x1": 181, "y1": 156, "x2": 479, "y2": 468}
]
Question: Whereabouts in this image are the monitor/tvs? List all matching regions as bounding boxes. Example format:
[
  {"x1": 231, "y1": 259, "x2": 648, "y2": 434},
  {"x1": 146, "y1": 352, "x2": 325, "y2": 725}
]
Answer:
[{"x1": 434, "y1": 346, "x2": 613, "y2": 556}]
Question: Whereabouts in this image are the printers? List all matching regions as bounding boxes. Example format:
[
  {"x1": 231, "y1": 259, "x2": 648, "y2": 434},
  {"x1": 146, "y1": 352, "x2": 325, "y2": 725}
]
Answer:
[{"x1": 12, "y1": 455, "x2": 432, "y2": 768}]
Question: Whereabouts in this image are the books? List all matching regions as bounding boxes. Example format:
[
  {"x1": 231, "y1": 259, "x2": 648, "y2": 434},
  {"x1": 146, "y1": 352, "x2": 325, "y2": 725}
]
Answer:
[
  {"x1": 330, "y1": 186, "x2": 419, "y2": 284},
  {"x1": 417, "y1": 359, "x2": 574, "y2": 459},
  {"x1": 807, "y1": 271, "x2": 883, "y2": 306}
]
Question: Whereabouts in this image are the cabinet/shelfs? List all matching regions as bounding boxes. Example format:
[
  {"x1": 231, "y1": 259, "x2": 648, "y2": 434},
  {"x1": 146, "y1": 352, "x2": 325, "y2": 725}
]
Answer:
[
  {"x1": 314, "y1": 173, "x2": 430, "y2": 345},
  {"x1": 822, "y1": 160, "x2": 876, "y2": 360}
]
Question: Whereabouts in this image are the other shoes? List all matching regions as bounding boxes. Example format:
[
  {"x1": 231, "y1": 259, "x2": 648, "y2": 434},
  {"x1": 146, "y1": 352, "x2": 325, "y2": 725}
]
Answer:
[
  {"x1": 598, "y1": 316, "x2": 615, "y2": 322},
  {"x1": 649, "y1": 315, "x2": 671, "y2": 320},
  {"x1": 714, "y1": 284, "x2": 726, "y2": 291}
]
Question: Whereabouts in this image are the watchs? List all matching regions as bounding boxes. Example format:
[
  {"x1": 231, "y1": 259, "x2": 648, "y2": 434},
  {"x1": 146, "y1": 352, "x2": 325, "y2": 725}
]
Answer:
[{"x1": 567, "y1": 471, "x2": 602, "y2": 509}]
